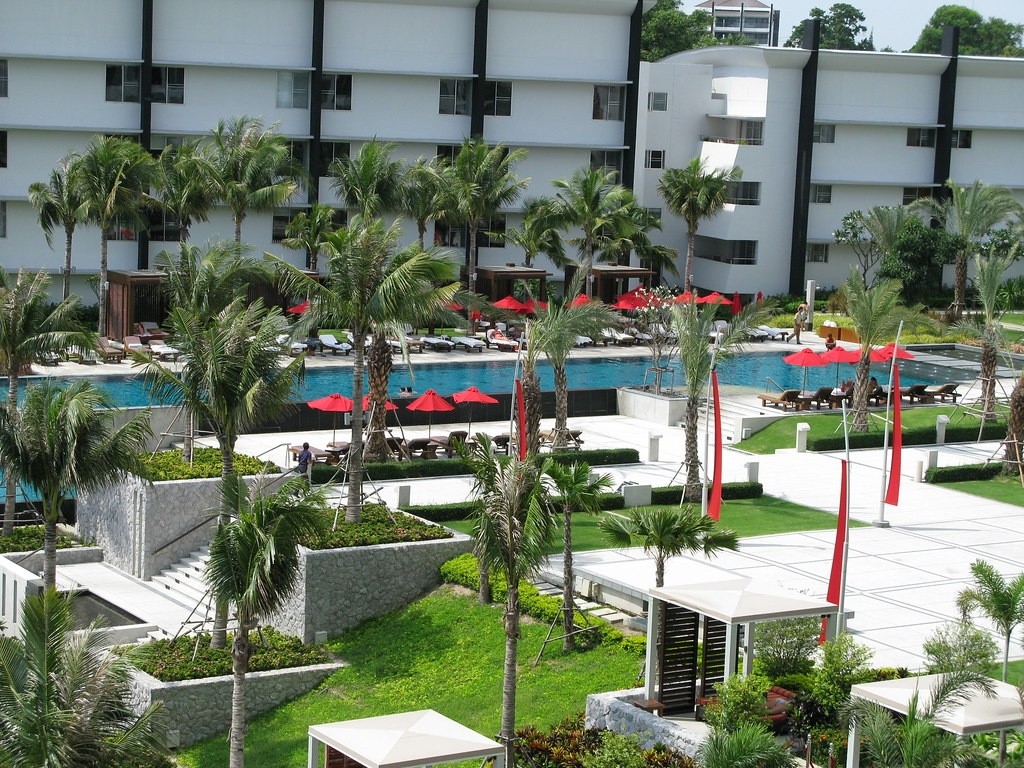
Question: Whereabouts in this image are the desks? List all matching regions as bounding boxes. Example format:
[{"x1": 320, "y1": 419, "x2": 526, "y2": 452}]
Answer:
[
  {"x1": 831, "y1": 394, "x2": 846, "y2": 408},
  {"x1": 797, "y1": 394, "x2": 813, "y2": 410}
]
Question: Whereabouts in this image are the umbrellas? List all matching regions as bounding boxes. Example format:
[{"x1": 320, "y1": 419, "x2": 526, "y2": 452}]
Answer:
[
  {"x1": 307, "y1": 393, "x2": 400, "y2": 450},
  {"x1": 757, "y1": 291, "x2": 763, "y2": 304},
  {"x1": 287, "y1": 301, "x2": 319, "y2": 314},
  {"x1": 406, "y1": 386, "x2": 499, "y2": 440},
  {"x1": 783, "y1": 341, "x2": 916, "y2": 397},
  {"x1": 444, "y1": 294, "x2": 592, "y2": 335},
  {"x1": 609, "y1": 284, "x2": 743, "y2": 317}
]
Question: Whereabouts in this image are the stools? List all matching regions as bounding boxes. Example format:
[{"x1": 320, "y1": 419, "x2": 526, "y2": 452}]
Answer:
[{"x1": 634, "y1": 700, "x2": 666, "y2": 718}]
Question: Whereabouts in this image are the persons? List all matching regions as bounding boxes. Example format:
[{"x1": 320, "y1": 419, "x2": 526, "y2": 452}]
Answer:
[
  {"x1": 825, "y1": 333, "x2": 836, "y2": 352},
  {"x1": 624, "y1": 320, "x2": 648, "y2": 344},
  {"x1": 786, "y1": 301, "x2": 808, "y2": 344},
  {"x1": 867, "y1": 377, "x2": 882, "y2": 406},
  {"x1": 293, "y1": 442, "x2": 313, "y2": 498},
  {"x1": 301, "y1": 335, "x2": 317, "y2": 356},
  {"x1": 490, "y1": 324, "x2": 508, "y2": 341},
  {"x1": 400, "y1": 386, "x2": 417, "y2": 397}
]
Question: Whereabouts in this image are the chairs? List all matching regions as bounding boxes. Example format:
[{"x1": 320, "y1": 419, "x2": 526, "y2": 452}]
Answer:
[
  {"x1": 325, "y1": 442, "x2": 364, "y2": 463},
  {"x1": 471, "y1": 433, "x2": 509, "y2": 456},
  {"x1": 540, "y1": 429, "x2": 582, "y2": 443},
  {"x1": 401, "y1": 438, "x2": 431, "y2": 460},
  {"x1": 868, "y1": 387, "x2": 882, "y2": 406},
  {"x1": 891, "y1": 384, "x2": 928, "y2": 404},
  {"x1": 387, "y1": 437, "x2": 404, "y2": 453},
  {"x1": 45, "y1": 320, "x2": 789, "y2": 363},
  {"x1": 804, "y1": 386, "x2": 834, "y2": 410},
  {"x1": 290, "y1": 445, "x2": 332, "y2": 467},
  {"x1": 925, "y1": 383, "x2": 962, "y2": 403},
  {"x1": 758, "y1": 390, "x2": 801, "y2": 412},
  {"x1": 431, "y1": 431, "x2": 467, "y2": 459}
]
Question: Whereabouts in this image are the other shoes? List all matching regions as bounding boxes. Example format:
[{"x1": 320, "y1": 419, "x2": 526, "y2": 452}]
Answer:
[
  {"x1": 293, "y1": 492, "x2": 300, "y2": 498},
  {"x1": 796, "y1": 342, "x2": 801, "y2": 344},
  {"x1": 787, "y1": 338, "x2": 789, "y2": 342}
]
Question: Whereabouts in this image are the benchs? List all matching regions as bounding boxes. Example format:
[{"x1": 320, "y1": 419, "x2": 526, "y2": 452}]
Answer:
[{"x1": 696, "y1": 686, "x2": 797, "y2": 735}]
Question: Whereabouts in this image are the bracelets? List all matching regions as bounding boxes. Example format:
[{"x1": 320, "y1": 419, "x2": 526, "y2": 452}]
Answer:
[{"x1": 310, "y1": 461, "x2": 312, "y2": 464}]
